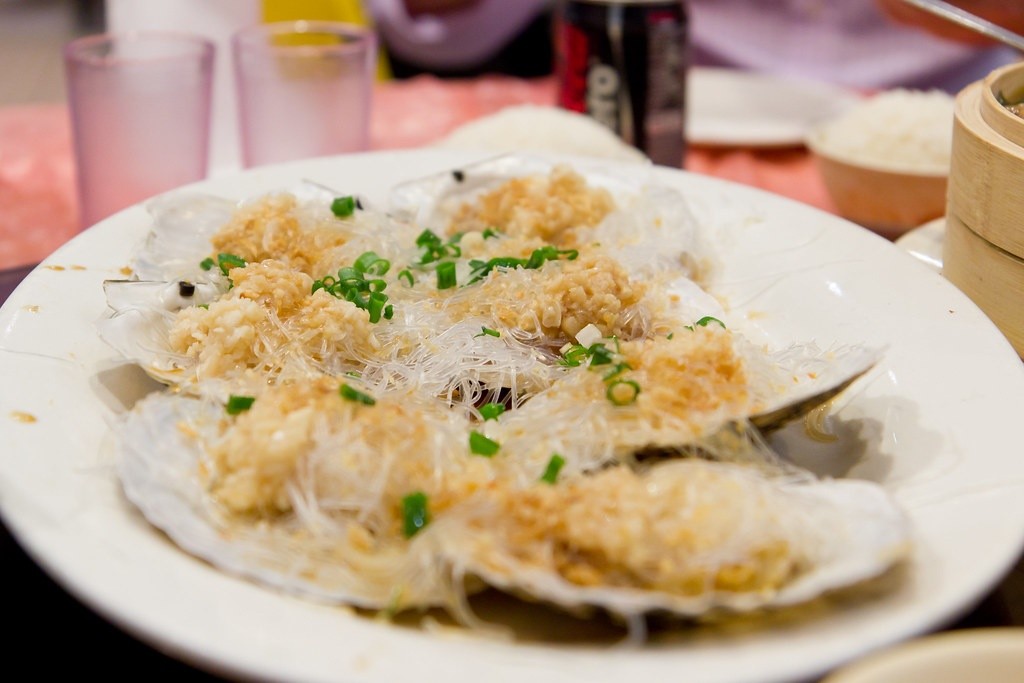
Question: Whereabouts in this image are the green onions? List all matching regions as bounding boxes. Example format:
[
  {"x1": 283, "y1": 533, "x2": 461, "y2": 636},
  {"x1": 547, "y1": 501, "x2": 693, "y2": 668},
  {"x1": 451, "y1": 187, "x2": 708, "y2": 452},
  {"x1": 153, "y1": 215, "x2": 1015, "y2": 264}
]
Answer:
[{"x1": 201, "y1": 194, "x2": 724, "y2": 533}]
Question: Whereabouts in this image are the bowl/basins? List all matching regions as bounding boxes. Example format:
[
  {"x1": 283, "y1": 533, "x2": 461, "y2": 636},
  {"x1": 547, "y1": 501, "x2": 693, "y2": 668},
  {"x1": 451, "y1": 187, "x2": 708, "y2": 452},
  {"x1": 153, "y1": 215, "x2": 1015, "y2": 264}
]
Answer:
[{"x1": 804, "y1": 134, "x2": 951, "y2": 246}]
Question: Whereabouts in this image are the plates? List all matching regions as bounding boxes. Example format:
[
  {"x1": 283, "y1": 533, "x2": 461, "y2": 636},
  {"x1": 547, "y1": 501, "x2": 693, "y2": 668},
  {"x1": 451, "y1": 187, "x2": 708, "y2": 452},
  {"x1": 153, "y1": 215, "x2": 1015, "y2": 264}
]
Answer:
[
  {"x1": 0, "y1": 136, "x2": 1024, "y2": 681},
  {"x1": 683, "y1": 62, "x2": 865, "y2": 149}
]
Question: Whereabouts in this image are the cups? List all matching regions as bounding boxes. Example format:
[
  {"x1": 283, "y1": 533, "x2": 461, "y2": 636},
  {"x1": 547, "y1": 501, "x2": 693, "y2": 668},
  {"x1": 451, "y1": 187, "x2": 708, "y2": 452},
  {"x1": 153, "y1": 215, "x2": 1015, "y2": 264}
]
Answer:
[
  {"x1": 229, "y1": 20, "x2": 373, "y2": 169},
  {"x1": 63, "y1": 27, "x2": 219, "y2": 234}
]
